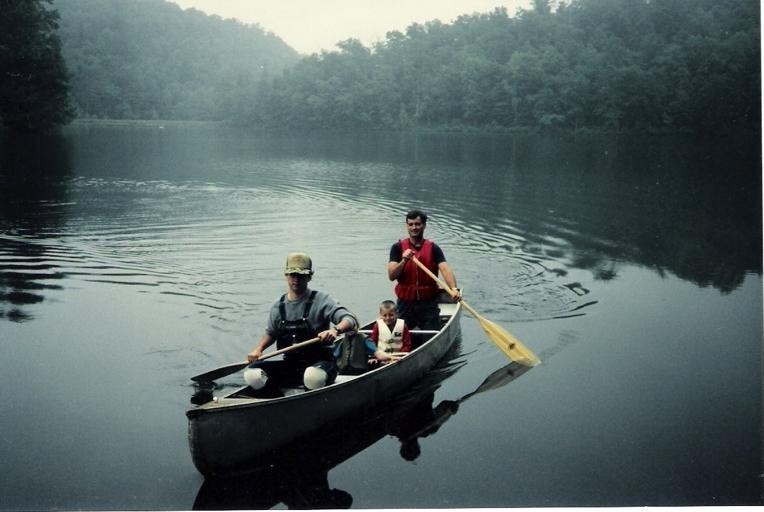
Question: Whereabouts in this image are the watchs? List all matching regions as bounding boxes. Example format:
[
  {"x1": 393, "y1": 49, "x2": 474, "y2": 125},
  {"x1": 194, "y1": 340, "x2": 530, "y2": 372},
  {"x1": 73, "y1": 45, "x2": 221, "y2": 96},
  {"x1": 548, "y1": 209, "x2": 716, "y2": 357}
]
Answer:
[{"x1": 333, "y1": 325, "x2": 342, "y2": 334}]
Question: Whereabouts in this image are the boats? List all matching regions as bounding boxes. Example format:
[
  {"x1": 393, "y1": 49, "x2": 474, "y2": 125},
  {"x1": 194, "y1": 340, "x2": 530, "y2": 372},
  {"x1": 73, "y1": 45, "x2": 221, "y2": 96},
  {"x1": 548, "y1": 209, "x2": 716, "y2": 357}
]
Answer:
[
  {"x1": 189, "y1": 332, "x2": 464, "y2": 509},
  {"x1": 186, "y1": 282, "x2": 463, "y2": 493}
]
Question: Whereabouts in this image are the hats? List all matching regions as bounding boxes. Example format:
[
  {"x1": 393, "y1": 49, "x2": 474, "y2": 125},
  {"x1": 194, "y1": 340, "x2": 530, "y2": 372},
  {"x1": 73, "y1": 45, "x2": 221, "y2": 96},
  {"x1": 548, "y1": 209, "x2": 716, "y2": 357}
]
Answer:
[{"x1": 283, "y1": 253, "x2": 313, "y2": 275}]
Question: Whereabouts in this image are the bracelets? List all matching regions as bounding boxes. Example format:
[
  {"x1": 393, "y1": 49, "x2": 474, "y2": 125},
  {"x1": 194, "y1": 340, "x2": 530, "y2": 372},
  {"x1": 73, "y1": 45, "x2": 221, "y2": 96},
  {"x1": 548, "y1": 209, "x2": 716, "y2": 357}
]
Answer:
[{"x1": 450, "y1": 287, "x2": 460, "y2": 292}]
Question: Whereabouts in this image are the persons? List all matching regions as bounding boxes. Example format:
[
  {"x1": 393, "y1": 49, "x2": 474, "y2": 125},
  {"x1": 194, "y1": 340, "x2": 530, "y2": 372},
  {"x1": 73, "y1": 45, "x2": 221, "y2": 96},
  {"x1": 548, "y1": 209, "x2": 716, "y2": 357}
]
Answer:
[
  {"x1": 333, "y1": 300, "x2": 412, "y2": 375},
  {"x1": 281, "y1": 472, "x2": 353, "y2": 510},
  {"x1": 388, "y1": 389, "x2": 459, "y2": 462},
  {"x1": 243, "y1": 250, "x2": 359, "y2": 391},
  {"x1": 387, "y1": 210, "x2": 462, "y2": 330}
]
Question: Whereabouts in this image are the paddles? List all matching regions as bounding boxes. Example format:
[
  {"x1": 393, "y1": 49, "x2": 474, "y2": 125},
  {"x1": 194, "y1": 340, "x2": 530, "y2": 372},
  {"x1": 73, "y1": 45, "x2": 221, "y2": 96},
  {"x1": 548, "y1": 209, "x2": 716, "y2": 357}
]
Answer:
[
  {"x1": 410, "y1": 253, "x2": 541, "y2": 360},
  {"x1": 189, "y1": 334, "x2": 321, "y2": 381}
]
